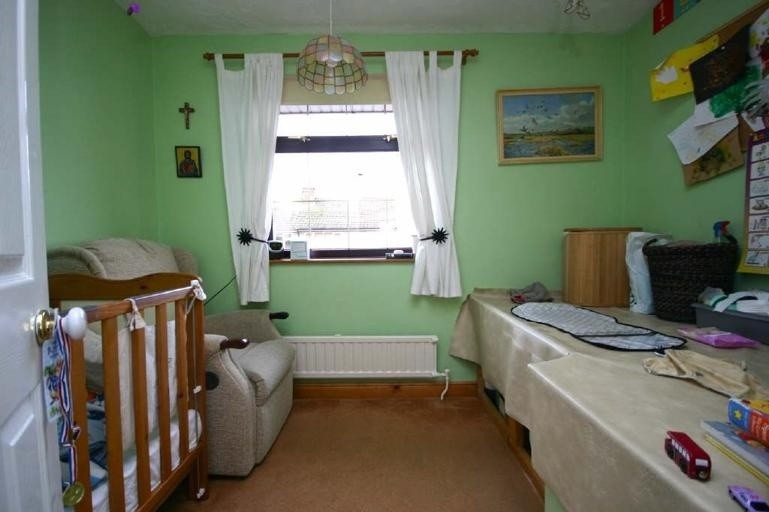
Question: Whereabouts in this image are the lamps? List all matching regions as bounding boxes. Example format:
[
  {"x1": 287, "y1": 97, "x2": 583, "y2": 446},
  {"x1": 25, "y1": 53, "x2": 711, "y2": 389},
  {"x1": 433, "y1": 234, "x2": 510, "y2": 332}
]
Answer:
[{"x1": 295, "y1": 0, "x2": 369, "y2": 96}]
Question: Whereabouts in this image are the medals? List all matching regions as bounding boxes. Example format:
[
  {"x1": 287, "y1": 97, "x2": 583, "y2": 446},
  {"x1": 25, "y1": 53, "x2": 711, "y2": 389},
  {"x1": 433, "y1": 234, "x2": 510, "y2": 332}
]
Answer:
[{"x1": 63, "y1": 481, "x2": 86, "y2": 508}]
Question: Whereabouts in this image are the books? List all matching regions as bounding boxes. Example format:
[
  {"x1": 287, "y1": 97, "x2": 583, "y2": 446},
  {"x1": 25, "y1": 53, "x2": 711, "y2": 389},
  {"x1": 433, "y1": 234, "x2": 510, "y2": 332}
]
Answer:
[
  {"x1": 728, "y1": 397, "x2": 769, "y2": 446},
  {"x1": 704, "y1": 433, "x2": 769, "y2": 487},
  {"x1": 700, "y1": 419, "x2": 769, "y2": 478}
]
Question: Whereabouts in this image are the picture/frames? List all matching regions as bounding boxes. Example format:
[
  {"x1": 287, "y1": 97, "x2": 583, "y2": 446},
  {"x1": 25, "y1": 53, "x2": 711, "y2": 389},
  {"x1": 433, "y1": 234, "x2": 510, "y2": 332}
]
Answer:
[
  {"x1": 175, "y1": 145, "x2": 202, "y2": 179},
  {"x1": 495, "y1": 85, "x2": 602, "y2": 165}
]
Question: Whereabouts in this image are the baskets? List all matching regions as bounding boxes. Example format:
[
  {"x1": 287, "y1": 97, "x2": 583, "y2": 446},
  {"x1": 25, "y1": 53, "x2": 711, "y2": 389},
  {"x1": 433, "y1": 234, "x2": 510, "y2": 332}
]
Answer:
[{"x1": 641, "y1": 234, "x2": 743, "y2": 324}]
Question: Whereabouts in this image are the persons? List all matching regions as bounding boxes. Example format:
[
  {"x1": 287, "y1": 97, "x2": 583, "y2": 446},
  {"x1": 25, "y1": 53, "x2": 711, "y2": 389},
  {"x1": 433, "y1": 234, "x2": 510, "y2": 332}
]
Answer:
[{"x1": 180, "y1": 151, "x2": 198, "y2": 175}]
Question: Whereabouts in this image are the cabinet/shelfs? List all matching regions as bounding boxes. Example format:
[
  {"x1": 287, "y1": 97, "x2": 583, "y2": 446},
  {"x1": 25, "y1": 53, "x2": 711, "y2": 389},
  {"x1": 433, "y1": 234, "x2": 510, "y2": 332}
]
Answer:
[{"x1": 564, "y1": 227, "x2": 641, "y2": 307}]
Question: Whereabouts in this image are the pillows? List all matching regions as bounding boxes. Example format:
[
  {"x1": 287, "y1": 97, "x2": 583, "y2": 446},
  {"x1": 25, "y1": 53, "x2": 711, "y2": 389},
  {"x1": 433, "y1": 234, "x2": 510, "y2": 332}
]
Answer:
[{"x1": 118, "y1": 319, "x2": 178, "y2": 453}]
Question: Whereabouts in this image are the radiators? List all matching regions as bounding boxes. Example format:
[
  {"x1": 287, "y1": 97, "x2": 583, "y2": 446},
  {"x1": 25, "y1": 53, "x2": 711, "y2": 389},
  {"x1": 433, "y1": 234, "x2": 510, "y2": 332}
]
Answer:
[{"x1": 285, "y1": 336, "x2": 450, "y2": 379}]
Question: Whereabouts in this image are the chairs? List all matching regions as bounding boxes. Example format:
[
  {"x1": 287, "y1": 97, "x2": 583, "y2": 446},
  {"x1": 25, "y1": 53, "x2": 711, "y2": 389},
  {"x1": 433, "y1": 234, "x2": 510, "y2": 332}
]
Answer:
[{"x1": 48, "y1": 237, "x2": 295, "y2": 478}]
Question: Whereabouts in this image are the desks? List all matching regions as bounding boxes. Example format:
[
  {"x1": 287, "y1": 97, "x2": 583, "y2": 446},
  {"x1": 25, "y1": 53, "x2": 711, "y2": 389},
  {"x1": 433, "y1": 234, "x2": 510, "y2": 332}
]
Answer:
[{"x1": 447, "y1": 293, "x2": 769, "y2": 512}]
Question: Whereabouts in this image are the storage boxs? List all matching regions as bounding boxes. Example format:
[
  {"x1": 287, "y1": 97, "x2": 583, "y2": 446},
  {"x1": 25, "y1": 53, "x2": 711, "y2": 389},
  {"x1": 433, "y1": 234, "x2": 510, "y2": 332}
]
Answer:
[{"x1": 690, "y1": 302, "x2": 769, "y2": 345}]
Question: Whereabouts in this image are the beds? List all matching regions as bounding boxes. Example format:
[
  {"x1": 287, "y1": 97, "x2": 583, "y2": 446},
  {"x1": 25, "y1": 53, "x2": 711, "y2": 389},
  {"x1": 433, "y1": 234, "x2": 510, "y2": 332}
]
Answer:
[{"x1": 48, "y1": 274, "x2": 209, "y2": 512}]
